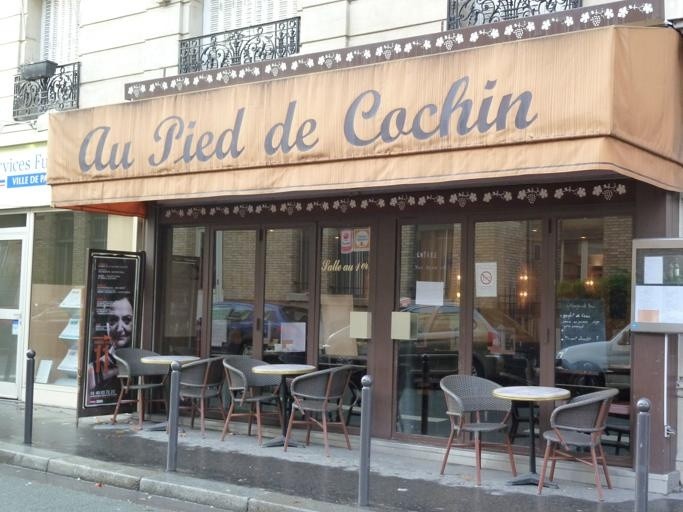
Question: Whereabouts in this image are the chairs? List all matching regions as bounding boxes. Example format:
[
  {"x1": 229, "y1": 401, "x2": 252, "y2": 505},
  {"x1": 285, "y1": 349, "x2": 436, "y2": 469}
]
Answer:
[
  {"x1": 440, "y1": 375, "x2": 517, "y2": 486},
  {"x1": 107, "y1": 348, "x2": 354, "y2": 457},
  {"x1": 538, "y1": 386, "x2": 619, "y2": 502}
]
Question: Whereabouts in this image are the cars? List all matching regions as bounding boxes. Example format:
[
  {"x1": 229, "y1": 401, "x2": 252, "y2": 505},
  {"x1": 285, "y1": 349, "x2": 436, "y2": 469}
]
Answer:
[
  {"x1": 196, "y1": 299, "x2": 308, "y2": 354},
  {"x1": 354, "y1": 299, "x2": 539, "y2": 391},
  {"x1": 555, "y1": 323, "x2": 631, "y2": 404}
]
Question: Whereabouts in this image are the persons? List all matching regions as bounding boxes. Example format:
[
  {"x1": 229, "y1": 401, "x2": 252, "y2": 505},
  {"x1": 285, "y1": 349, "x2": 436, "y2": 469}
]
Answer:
[
  {"x1": 219, "y1": 330, "x2": 243, "y2": 354},
  {"x1": 87, "y1": 287, "x2": 132, "y2": 402}
]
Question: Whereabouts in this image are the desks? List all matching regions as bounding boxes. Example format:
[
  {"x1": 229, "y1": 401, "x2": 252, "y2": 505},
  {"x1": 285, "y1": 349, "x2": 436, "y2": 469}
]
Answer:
[{"x1": 491, "y1": 386, "x2": 571, "y2": 488}]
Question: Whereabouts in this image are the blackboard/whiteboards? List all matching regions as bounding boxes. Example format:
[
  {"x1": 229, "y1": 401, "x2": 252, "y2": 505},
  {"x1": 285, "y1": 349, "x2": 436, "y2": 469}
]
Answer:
[{"x1": 560, "y1": 298, "x2": 608, "y2": 374}]
